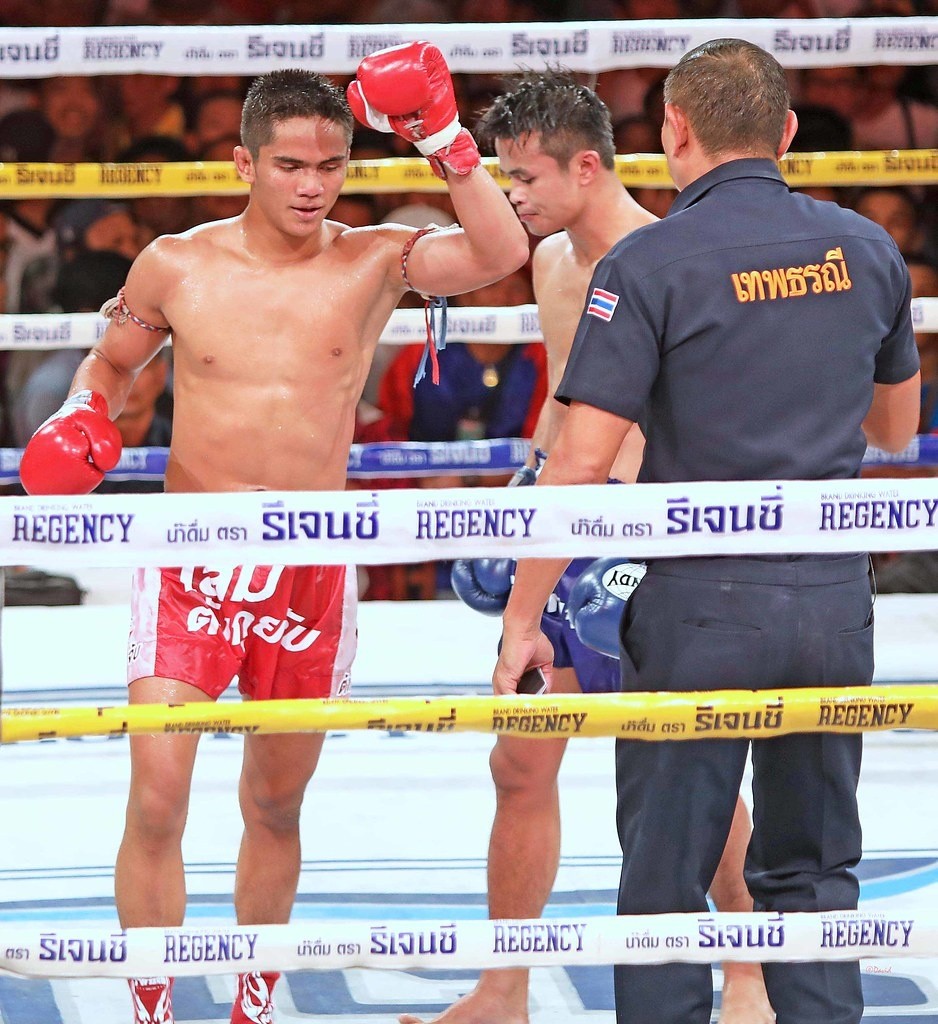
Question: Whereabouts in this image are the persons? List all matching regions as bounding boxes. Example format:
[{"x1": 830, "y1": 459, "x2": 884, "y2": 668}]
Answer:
[
  {"x1": 450, "y1": 60, "x2": 776, "y2": 1024},
  {"x1": 493, "y1": 37, "x2": 920, "y2": 1024},
  {"x1": 0, "y1": 0, "x2": 938, "y2": 602},
  {"x1": 17, "y1": 40, "x2": 529, "y2": 1024}
]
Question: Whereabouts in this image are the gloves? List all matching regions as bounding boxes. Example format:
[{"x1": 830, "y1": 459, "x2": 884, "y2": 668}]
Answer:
[
  {"x1": 19, "y1": 389, "x2": 122, "y2": 495},
  {"x1": 451, "y1": 468, "x2": 537, "y2": 617},
  {"x1": 346, "y1": 41, "x2": 482, "y2": 180},
  {"x1": 566, "y1": 558, "x2": 647, "y2": 659}
]
